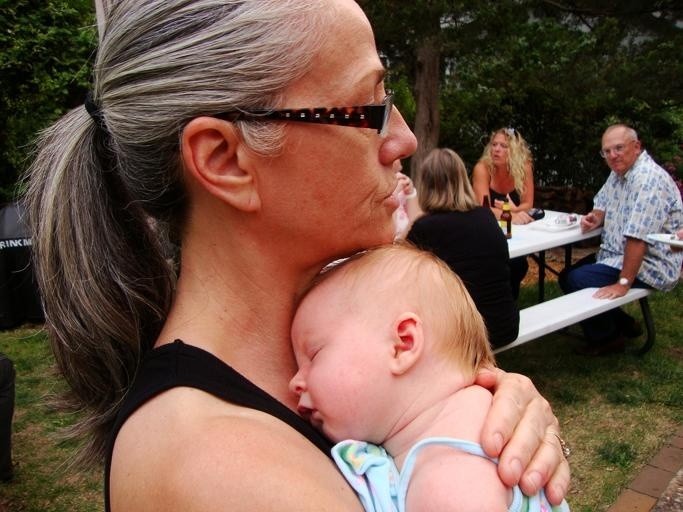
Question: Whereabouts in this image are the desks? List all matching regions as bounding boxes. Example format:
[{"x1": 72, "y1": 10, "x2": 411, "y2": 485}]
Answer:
[{"x1": 495, "y1": 207, "x2": 606, "y2": 302}]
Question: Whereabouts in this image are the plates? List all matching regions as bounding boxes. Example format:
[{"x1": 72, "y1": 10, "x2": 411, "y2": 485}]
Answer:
[
  {"x1": 530, "y1": 213, "x2": 580, "y2": 232},
  {"x1": 646, "y1": 233, "x2": 682, "y2": 248}
]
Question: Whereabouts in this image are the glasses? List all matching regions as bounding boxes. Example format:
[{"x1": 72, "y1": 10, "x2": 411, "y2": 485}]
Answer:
[
  {"x1": 212, "y1": 88, "x2": 396, "y2": 142},
  {"x1": 600, "y1": 139, "x2": 636, "y2": 158}
]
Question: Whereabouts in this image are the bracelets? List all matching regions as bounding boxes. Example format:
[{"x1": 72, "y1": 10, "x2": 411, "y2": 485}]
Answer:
[{"x1": 405, "y1": 187, "x2": 417, "y2": 200}]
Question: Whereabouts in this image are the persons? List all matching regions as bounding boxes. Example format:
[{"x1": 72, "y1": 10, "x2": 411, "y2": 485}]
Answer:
[
  {"x1": 473, "y1": 127, "x2": 535, "y2": 224},
  {"x1": 393, "y1": 158, "x2": 424, "y2": 244},
  {"x1": 558, "y1": 124, "x2": 683, "y2": 342},
  {"x1": 288, "y1": 239, "x2": 570, "y2": 512},
  {"x1": 405, "y1": 147, "x2": 520, "y2": 351},
  {"x1": 18, "y1": 0, "x2": 571, "y2": 512}
]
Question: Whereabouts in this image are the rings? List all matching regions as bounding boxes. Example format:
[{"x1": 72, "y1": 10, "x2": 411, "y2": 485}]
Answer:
[{"x1": 546, "y1": 432, "x2": 570, "y2": 459}]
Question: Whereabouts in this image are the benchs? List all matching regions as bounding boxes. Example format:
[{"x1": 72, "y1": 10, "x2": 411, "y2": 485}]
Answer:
[{"x1": 491, "y1": 286, "x2": 660, "y2": 355}]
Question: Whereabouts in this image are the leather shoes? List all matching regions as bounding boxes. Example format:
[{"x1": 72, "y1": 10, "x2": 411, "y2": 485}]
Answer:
[
  {"x1": 574, "y1": 336, "x2": 626, "y2": 356},
  {"x1": 621, "y1": 320, "x2": 644, "y2": 339}
]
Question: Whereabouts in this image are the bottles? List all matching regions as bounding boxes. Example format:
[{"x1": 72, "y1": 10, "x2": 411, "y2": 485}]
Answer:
[{"x1": 500, "y1": 206, "x2": 511, "y2": 238}]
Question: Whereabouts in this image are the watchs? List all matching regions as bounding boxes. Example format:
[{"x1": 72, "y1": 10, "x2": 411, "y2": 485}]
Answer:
[
  {"x1": 617, "y1": 277, "x2": 630, "y2": 286},
  {"x1": 500, "y1": 211, "x2": 512, "y2": 222}
]
